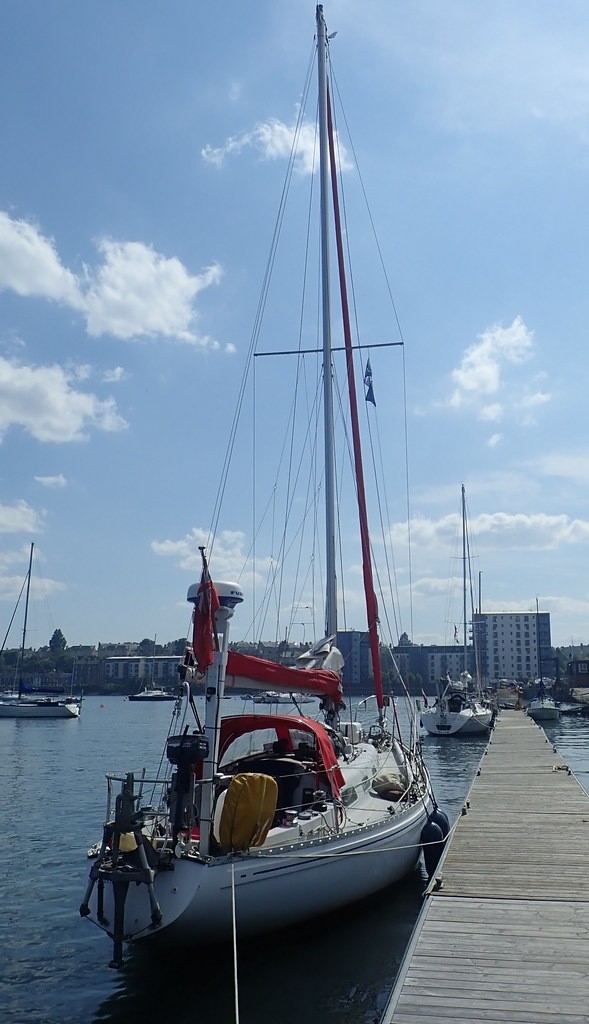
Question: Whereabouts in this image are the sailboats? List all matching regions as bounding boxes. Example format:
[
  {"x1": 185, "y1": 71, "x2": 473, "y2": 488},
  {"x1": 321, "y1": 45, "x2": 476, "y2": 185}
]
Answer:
[
  {"x1": 253, "y1": 626, "x2": 316, "y2": 703},
  {"x1": 128, "y1": 633, "x2": 182, "y2": 701},
  {"x1": 0, "y1": 541, "x2": 81, "y2": 719},
  {"x1": 419, "y1": 482, "x2": 494, "y2": 737},
  {"x1": 527, "y1": 598, "x2": 560, "y2": 721},
  {"x1": 77, "y1": 4, "x2": 438, "y2": 958}
]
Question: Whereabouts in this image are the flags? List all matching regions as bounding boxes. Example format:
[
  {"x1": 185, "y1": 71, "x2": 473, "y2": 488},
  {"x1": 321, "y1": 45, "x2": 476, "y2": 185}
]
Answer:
[
  {"x1": 193, "y1": 562, "x2": 220, "y2": 675},
  {"x1": 364, "y1": 356, "x2": 376, "y2": 408}
]
